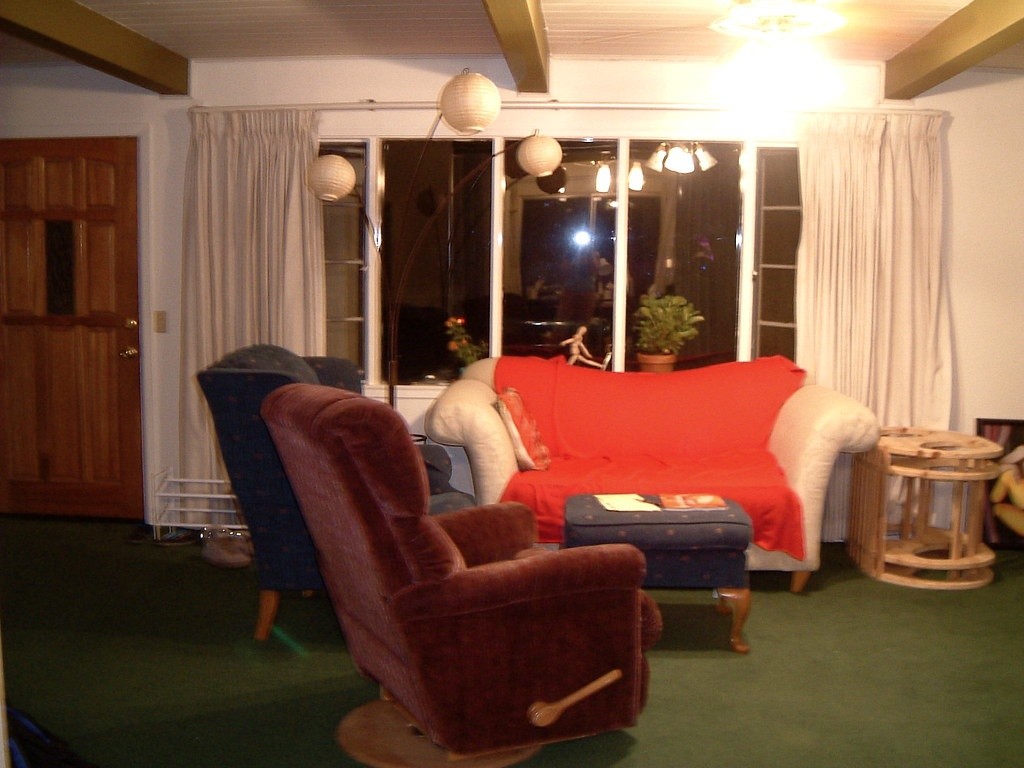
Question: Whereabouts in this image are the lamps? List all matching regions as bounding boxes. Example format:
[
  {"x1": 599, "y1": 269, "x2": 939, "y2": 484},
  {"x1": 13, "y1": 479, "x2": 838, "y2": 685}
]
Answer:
[
  {"x1": 417, "y1": 145, "x2": 567, "y2": 313},
  {"x1": 628, "y1": 161, "x2": 643, "y2": 191},
  {"x1": 644, "y1": 142, "x2": 718, "y2": 174},
  {"x1": 596, "y1": 161, "x2": 611, "y2": 193},
  {"x1": 308, "y1": 68, "x2": 561, "y2": 442}
]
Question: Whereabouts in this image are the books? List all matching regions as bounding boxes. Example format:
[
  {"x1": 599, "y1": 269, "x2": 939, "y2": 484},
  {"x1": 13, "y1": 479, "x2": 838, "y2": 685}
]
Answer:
[{"x1": 660, "y1": 493, "x2": 730, "y2": 511}]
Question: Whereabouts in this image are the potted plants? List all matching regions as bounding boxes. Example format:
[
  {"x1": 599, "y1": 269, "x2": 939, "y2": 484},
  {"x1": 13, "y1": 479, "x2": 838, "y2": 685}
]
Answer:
[{"x1": 633, "y1": 292, "x2": 705, "y2": 372}]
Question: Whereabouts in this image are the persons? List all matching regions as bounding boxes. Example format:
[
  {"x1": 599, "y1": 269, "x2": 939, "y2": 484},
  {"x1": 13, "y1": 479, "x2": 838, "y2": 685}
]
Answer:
[{"x1": 672, "y1": 237, "x2": 739, "y2": 322}]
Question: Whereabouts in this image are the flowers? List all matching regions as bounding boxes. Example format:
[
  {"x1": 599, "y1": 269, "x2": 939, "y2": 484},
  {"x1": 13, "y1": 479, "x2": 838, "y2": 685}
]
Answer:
[{"x1": 444, "y1": 317, "x2": 489, "y2": 367}]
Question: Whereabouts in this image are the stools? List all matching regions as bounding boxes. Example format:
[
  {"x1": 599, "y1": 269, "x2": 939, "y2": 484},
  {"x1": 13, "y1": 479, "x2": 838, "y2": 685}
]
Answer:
[{"x1": 565, "y1": 493, "x2": 751, "y2": 653}]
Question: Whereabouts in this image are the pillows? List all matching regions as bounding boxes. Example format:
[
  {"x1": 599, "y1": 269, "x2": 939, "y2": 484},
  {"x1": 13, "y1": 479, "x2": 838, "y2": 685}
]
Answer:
[{"x1": 496, "y1": 388, "x2": 551, "y2": 471}]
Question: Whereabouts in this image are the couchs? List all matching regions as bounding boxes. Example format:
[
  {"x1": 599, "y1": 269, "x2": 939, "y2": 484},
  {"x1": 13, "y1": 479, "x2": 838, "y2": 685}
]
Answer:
[
  {"x1": 260, "y1": 382, "x2": 662, "y2": 768},
  {"x1": 424, "y1": 354, "x2": 880, "y2": 590},
  {"x1": 197, "y1": 344, "x2": 474, "y2": 640}
]
